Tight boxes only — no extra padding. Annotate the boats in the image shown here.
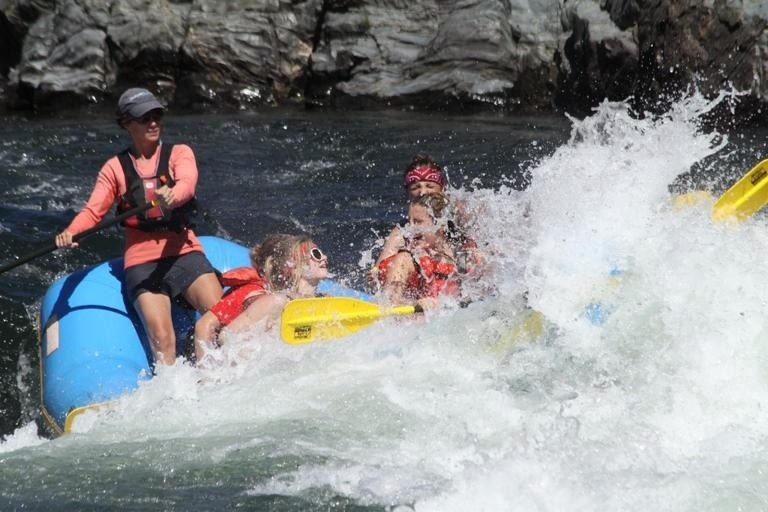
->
[36,236,379,444]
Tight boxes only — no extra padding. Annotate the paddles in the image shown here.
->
[710,156,768,230]
[280,297,483,346]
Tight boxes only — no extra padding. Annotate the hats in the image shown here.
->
[117,87,165,125]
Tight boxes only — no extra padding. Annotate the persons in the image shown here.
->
[55,87,224,366]
[372,153,486,315]
[193,234,334,361]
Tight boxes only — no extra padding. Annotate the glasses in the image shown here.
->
[309,248,324,262]
[129,109,164,125]
